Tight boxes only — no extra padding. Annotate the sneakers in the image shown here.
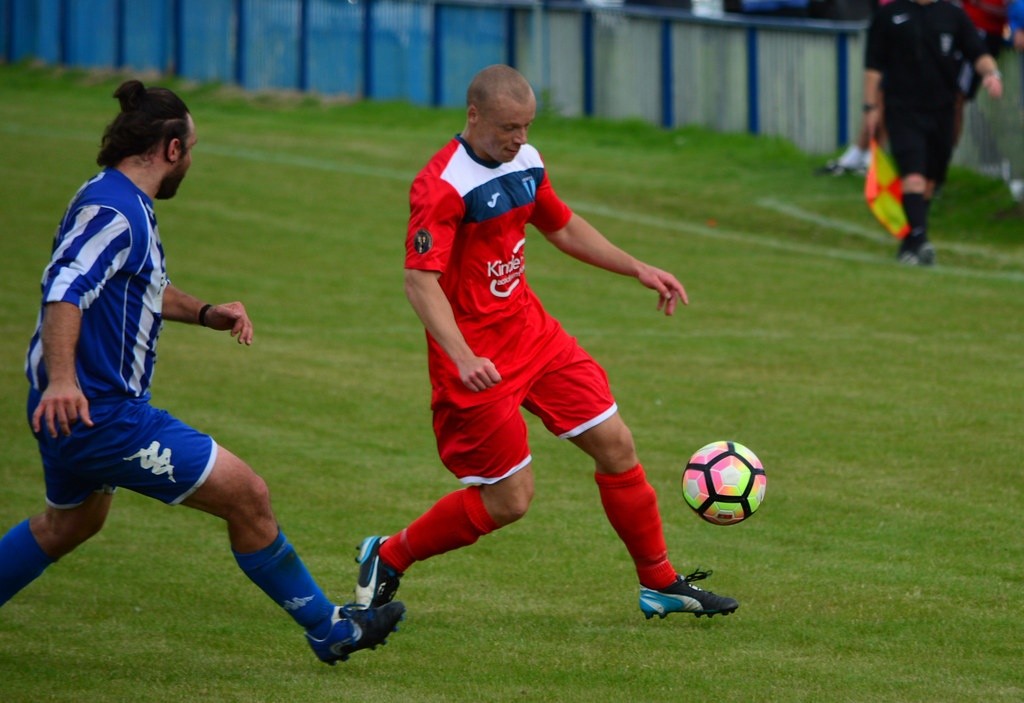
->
[354,536,403,610]
[637,574,740,619]
[304,600,408,667]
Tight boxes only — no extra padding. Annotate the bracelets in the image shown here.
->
[863,105,873,111]
[199,305,212,326]
[993,70,1002,79]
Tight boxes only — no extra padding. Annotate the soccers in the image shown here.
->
[681,438,768,526]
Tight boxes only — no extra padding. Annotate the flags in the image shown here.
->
[865,139,911,239]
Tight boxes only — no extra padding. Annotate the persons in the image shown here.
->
[810,0,1024,221]
[0,80,409,666]
[352,64,738,619]
[864,0,1002,265]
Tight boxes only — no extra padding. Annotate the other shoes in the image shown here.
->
[812,156,858,178]
[896,241,935,268]
